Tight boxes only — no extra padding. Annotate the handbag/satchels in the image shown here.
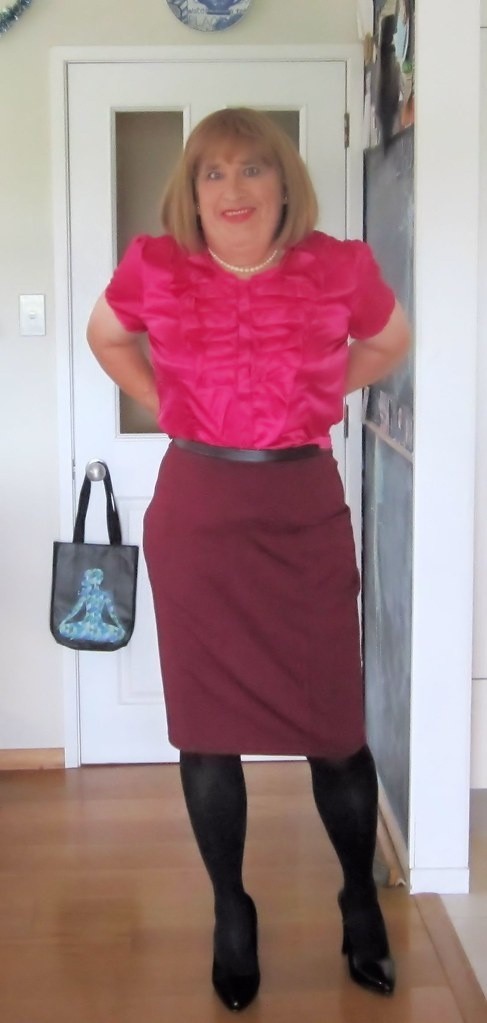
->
[51,463,139,651]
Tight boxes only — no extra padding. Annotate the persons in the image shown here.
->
[86,106,410,1012]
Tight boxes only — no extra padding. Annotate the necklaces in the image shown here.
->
[207,245,278,273]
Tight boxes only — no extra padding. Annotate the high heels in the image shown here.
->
[337,887,394,994]
[212,893,260,1013]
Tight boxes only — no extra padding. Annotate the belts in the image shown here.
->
[172,438,332,462]
